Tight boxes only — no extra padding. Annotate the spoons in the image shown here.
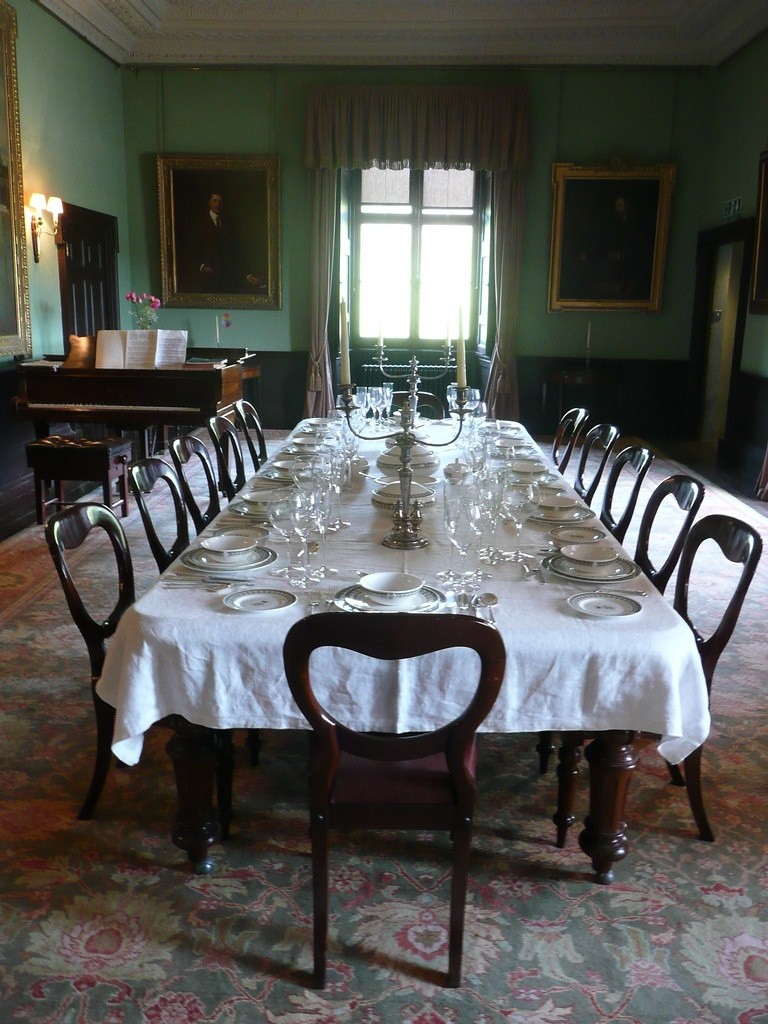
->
[481,592,499,624]
[456,590,469,615]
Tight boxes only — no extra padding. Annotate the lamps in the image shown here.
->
[30,193,64,263]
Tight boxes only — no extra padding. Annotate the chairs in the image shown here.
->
[283,612,507,991]
[44,501,261,840]
[533,407,762,849]
[375,391,445,420]
[127,400,268,575]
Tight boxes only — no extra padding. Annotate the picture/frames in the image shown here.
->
[748,150,768,316]
[155,151,282,310]
[0,0,33,362]
[547,161,675,314]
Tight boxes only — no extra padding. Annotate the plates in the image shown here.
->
[281,446,318,456]
[494,421,568,495]
[334,584,447,612]
[567,591,645,619]
[222,587,299,614]
[178,546,280,578]
[226,501,270,522]
[261,468,309,482]
[241,488,294,512]
[529,506,597,528]
[299,426,320,435]
[541,554,642,584]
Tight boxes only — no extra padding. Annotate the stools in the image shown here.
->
[26,434,135,525]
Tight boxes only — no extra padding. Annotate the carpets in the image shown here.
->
[1,432,768,1024]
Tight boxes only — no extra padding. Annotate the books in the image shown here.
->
[183,357,228,368]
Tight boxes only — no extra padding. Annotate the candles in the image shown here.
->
[586,320,591,348]
[341,296,351,385]
[444,332,451,346]
[378,331,383,346]
[216,315,219,343]
[456,303,466,388]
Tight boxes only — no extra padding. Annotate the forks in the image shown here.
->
[326,584,339,612]
[471,594,484,620]
[573,583,647,596]
[309,583,322,615]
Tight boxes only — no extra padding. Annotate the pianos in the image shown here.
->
[14,346,256,499]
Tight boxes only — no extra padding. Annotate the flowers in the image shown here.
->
[125,291,161,330]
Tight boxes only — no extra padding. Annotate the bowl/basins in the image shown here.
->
[560,544,622,574]
[374,474,441,486]
[510,462,548,482]
[527,494,579,519]
[358,571,425,606]
[548,524,607,550]
[200,535,258,563]
[307,417,328,431]
[214,525,271,548]
[292,438,324,452]
[272,459,310,477]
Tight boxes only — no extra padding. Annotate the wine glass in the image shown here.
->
[267,488,307,578]
[434,382,542,593]
[288,382,393,589]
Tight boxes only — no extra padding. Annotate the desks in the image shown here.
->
[242,364,262,425]
[96,418,711,885]
[542,368,628,438]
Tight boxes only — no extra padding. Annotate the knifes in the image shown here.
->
[164,573,254,582]
